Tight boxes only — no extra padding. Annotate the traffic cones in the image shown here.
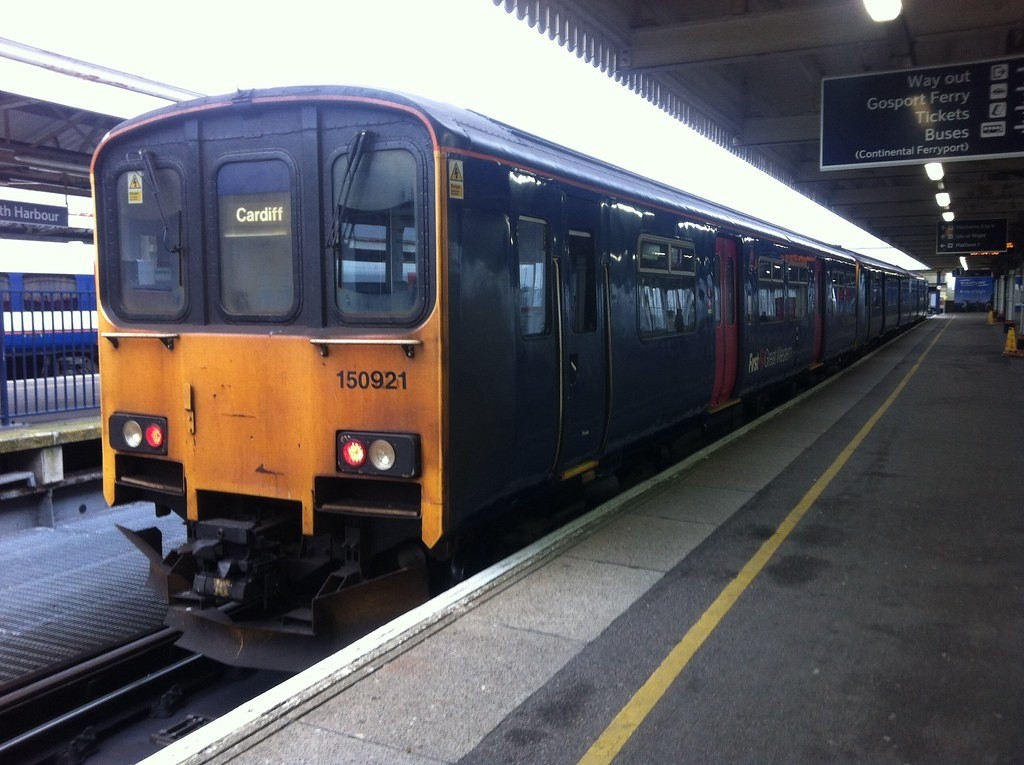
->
[1002,327,1018,357]
[987,310,994,325]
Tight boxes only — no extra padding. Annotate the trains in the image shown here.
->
[90,82,929,676]
